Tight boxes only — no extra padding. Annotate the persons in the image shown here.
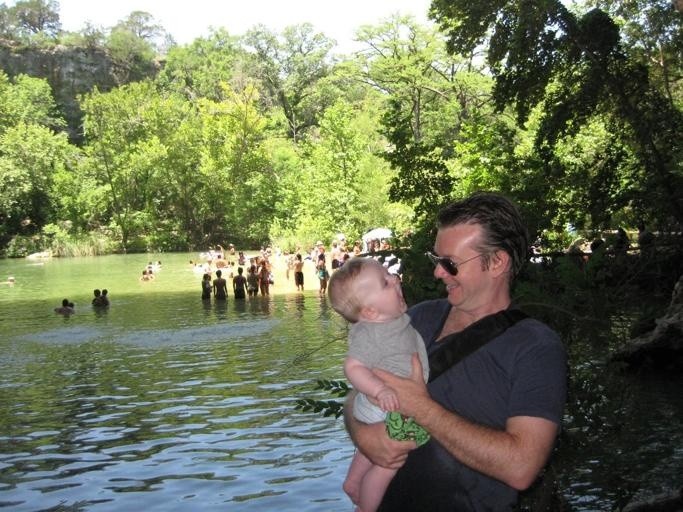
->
[341,191,569,512]
[561,224,666,277]
[325,255,429,511]
[54,298,75,315]
[139,231,410,301]
[92,289,108,307]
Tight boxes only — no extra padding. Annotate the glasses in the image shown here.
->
[426,252,485,276]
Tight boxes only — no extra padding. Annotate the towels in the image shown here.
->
[385,413,431,449]
